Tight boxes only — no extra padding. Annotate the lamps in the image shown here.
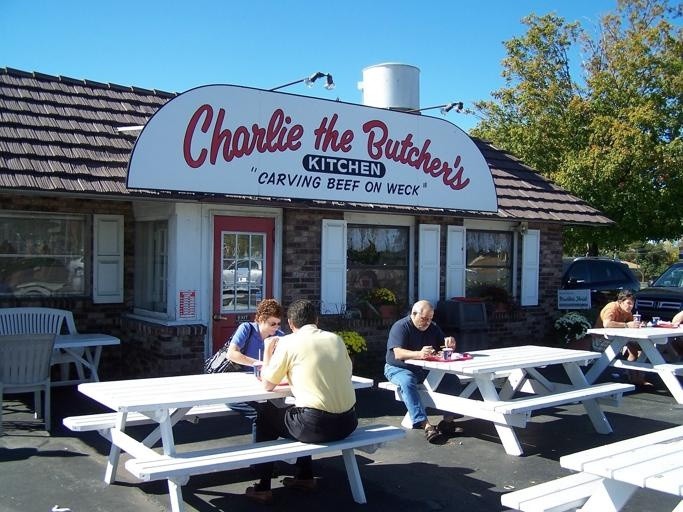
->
[404,100,466,116]
[268,68,337,94]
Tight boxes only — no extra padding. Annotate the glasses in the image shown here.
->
[269,322,282,326]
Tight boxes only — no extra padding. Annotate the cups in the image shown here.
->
[632,314,641,322]
[653,317,659,324]
[252,361,261,377]
[442,347,452,359]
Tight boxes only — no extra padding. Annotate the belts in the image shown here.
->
[304,406,356,419]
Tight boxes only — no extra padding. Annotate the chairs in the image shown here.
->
[0,330,59,434]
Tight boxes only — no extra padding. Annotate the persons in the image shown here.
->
[223,299,298,477]
[245,298,358,501]
[672,311,683,358]
[592,291,646,385]
[384,300,464,444]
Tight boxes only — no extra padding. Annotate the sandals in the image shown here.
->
[424,426,439,442]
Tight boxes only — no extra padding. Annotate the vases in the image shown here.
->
[347,349,358,370]
[367,303,399,321]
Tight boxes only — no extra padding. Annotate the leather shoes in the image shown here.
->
[245,487,272,504]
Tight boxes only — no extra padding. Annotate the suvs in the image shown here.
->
[561,257,640,312]
[629,262,682,354]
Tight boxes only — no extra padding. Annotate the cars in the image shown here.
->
[221,257,263,293]
[346,264,403,293]
[0,241,84,297]
[467,250,512,294]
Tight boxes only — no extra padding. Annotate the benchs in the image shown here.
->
[62,394,297,486]
[123,421,407,512]
[378,366,569,430]
[494,471,612,509]
[488,382,646,457]
[0,306,99,387]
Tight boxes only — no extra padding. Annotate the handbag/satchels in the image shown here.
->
[203,337,241,373]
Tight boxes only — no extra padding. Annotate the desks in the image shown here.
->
[554,420,682,508]
[398,343,616,457]
[76,366,374,512]
[49,333,120,395]
[571,326,681,406]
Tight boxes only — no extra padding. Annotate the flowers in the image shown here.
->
[331,330,367,353]
[368,286,400,305]
[553,311,592,343]
